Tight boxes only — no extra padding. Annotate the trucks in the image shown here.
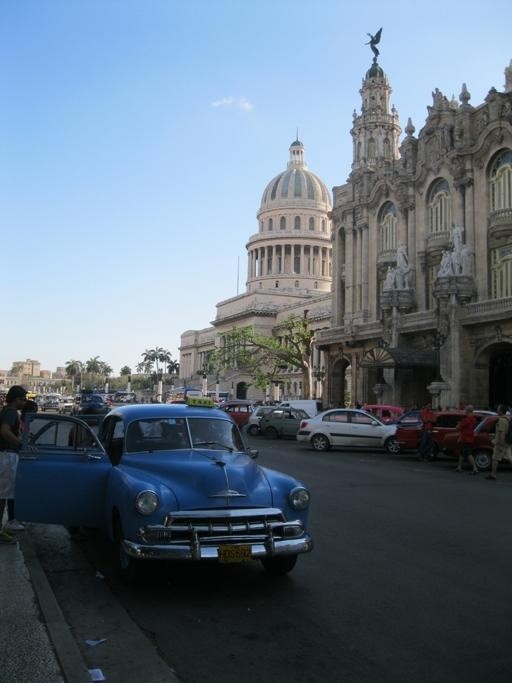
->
[259,398,323,439]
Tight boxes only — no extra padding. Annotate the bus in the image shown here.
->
[186,390,229,401]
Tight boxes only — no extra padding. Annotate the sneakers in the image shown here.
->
[1,519,23,542]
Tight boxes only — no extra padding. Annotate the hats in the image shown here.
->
[6,385,27,402]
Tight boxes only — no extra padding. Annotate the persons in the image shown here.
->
[384,223,475,290]
[364,26,384,58]
[404,116,416,137]
[254,398,512,482]
[432,86,443,110]
[17,401,38,527]
[1,384,27,544]
[459,83,471,106]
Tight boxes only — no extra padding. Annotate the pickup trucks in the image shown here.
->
[393,411,497,460]
[325,404,422,426]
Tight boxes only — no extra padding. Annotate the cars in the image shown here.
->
[297,408,402,455]
[167,400,186,404]
[216,399,255,428]
[14,396,314,577]
[0,387,136,413]
[244,405,276,435]
[259,407,311,439]
[442,413,512,471]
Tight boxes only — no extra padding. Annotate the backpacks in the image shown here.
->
[502,416,512,443]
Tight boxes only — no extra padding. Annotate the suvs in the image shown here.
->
[70,392,112,426]
